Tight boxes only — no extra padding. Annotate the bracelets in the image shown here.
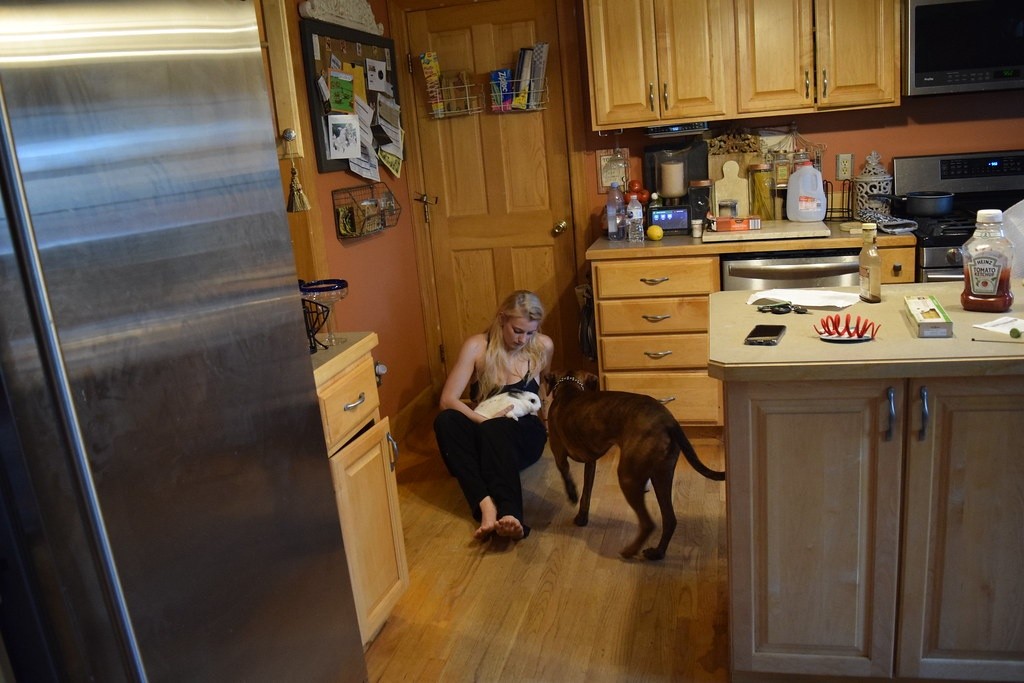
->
[543,419,548,421]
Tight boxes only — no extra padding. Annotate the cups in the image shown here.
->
[654,146,690,199]
[691,219,703,238]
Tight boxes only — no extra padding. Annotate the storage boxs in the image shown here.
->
[903,295,953,337]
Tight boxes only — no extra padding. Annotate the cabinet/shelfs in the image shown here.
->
[709,286,1024,682]
[733,1,894,114]
[588,0,724,124]
[594,253,719,430]
[315,351,408,642]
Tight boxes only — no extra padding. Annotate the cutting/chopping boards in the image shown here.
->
[701,219,831,242]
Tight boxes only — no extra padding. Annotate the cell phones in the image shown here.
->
[744,324,787,346]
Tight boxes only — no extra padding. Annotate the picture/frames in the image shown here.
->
[595,147,631,194]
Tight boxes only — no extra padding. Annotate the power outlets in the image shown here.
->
[837,153,854,181]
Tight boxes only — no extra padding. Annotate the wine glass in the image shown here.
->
[298,278,349,346]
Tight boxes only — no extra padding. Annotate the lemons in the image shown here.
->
[646,225,664,241]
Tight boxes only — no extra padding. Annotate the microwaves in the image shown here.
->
[901,0,1024,99]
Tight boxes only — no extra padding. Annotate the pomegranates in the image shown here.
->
[624,180,649,202]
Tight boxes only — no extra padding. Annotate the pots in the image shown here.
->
[868,191,955,217]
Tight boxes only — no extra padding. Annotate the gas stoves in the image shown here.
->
[892,149,1024,268]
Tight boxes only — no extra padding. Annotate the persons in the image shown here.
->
[433,289,554,540]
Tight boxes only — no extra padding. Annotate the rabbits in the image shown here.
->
[474,388,542,422]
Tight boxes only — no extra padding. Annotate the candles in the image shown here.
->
[661,161,685,197]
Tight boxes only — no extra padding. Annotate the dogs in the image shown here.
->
[544,368,729,561]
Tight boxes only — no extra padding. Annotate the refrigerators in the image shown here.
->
[0,3,370,681]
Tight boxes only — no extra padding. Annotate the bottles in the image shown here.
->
[626,195,644,243]
[748,163,777,219]
[859,223,882,304]
[961,209,1014,313]
[772,149,810,188]
[607,182,626,241]
[786,162,827,223]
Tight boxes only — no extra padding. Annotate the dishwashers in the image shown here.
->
[720,253,859,290]
